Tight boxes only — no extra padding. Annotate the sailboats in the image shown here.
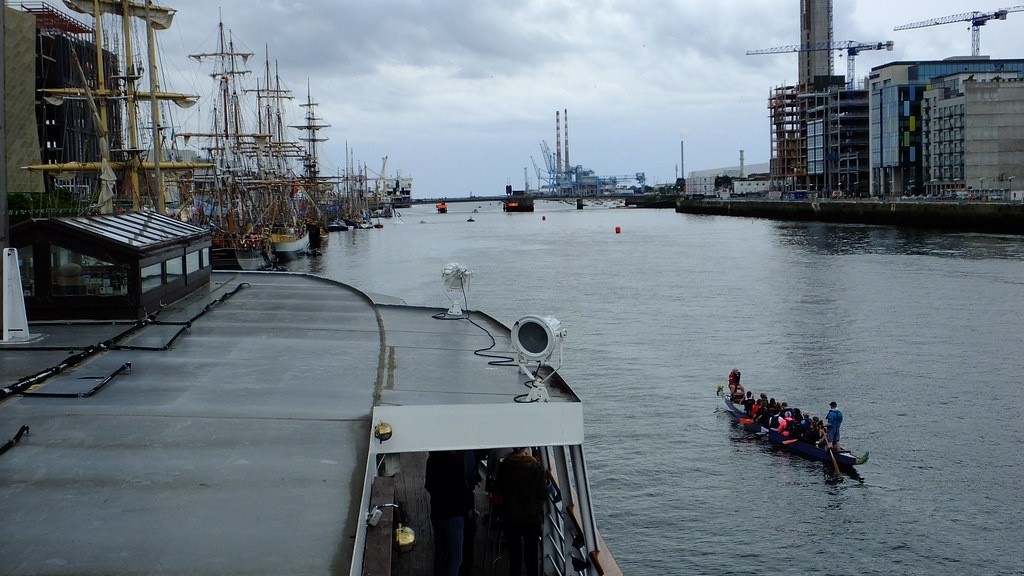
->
[19,0,415,271]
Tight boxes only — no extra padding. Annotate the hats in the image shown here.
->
[829,402,837,407]
[732,367,739,371]
[803,413,809,416]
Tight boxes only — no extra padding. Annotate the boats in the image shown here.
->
[715,385,869,473]
[498,189,536,213]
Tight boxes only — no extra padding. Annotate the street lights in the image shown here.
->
[1008,176,1017,202]
[980,177,987,198]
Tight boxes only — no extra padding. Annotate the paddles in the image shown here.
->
[781,438,799,444]
[822,428,840,473]
[739,418,757,425]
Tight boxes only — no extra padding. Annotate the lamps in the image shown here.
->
[441,263,473,315]
[511,315,568,403]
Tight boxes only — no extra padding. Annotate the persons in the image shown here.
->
[728,368,745,401]
[739,390,825,449]
[825,402,843,450]
[423,450,483,576]
[212,230,263,248]
[491,447,550,576]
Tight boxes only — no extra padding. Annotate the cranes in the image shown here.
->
[746,39,895,91]
[893,5,1024,57]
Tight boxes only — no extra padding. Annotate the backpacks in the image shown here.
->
[783,419,795,432]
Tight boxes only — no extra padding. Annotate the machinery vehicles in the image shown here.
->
[529,140,647,198]
